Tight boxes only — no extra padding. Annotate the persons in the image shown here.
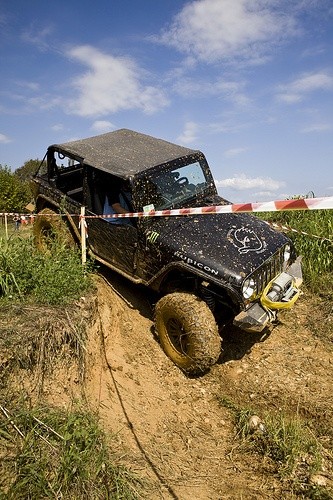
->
[102,176,134,228]
[13,215,19,231]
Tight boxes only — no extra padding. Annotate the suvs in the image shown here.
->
[29,127,305,379]
